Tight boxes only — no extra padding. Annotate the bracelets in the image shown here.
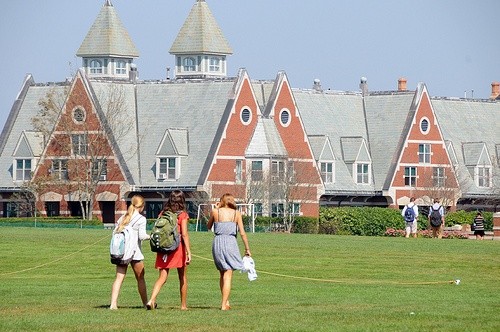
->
[245,248,249,251]
[187,251,191,255]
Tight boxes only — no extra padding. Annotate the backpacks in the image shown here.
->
[149,206,184,253]
[405,204,415,222]
[109,212,143,267]
[430,205,442,227]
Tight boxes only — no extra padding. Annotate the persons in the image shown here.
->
[145,190,192,311]
[401,197,419,238]
[108,195,158,311]
[207,193,252,311]
[427,198,444,239]
[474,210,485,240]
